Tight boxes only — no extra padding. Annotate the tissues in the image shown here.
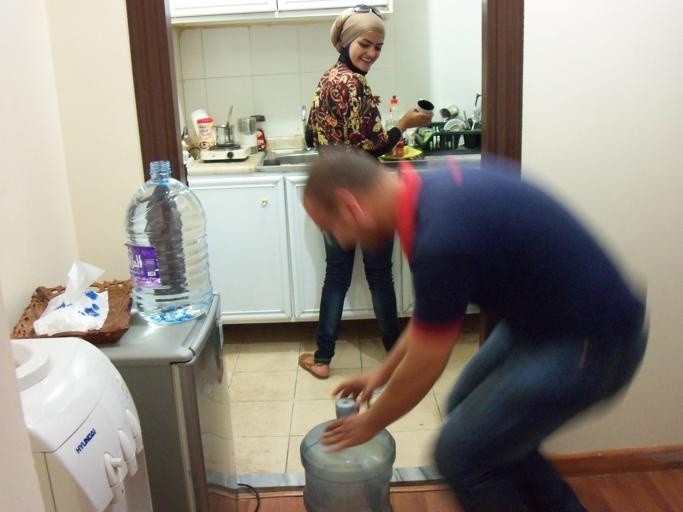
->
[31,257,109,336]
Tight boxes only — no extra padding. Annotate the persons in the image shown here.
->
[298,4,434,379]
[300,146,648,511]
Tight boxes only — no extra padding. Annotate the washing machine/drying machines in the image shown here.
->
[11,335,155,512]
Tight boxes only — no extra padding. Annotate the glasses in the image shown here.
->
[348,4,385,20]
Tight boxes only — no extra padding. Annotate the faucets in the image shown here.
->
[300,105,312,152]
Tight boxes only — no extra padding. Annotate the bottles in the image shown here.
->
[385,96,401,131]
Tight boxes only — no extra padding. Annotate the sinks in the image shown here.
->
[254,147,320,171]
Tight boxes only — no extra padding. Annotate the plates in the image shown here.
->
[380,145,422,161]
[444,119,469,132]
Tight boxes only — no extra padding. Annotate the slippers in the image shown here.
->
[298,353,329,379]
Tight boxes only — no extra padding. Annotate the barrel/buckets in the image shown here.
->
[122,158,215,324]
[298,397,397,512]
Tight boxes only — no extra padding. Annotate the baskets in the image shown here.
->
[11,278,133,346]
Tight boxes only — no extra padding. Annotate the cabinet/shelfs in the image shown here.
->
[169,0,394,29]
[285,173,481,322]
[188,175,293,325]
